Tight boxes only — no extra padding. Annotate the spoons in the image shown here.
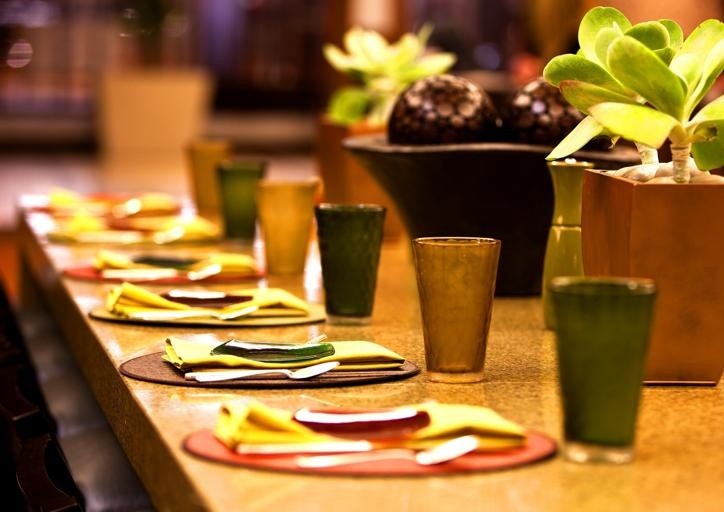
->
[125,305,260,322]
[296,433,479,468]
[195,359,339,383]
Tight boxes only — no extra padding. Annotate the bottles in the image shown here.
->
[540,160,594,331]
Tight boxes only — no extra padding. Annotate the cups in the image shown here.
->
[256,177,320,274]
[548,276,658,461]
[413,237,502,382]
[215,155,267,239]
[187,140,227,217]
[314,203,388,323]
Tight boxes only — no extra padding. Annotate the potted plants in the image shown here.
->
[316,27,453,242]
[546,6,724,387]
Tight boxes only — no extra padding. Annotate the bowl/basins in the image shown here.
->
[340,133,643,298]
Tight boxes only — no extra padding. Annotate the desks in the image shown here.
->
[1,192,724,512]
